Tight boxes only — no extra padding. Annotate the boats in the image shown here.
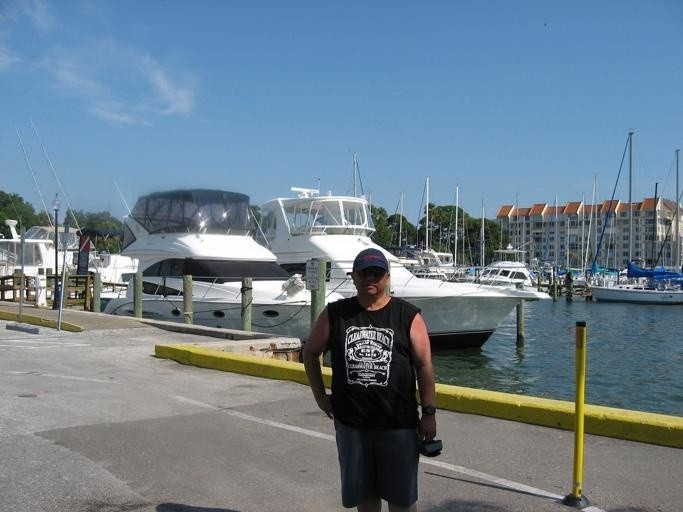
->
[0,218,137,301]
[103,191,345,345]
[258,194,538,346]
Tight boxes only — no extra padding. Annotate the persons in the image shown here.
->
[301,247,436,512]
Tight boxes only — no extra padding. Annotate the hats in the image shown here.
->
[353,249,388,272]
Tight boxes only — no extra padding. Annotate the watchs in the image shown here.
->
[421,405,436,416]
